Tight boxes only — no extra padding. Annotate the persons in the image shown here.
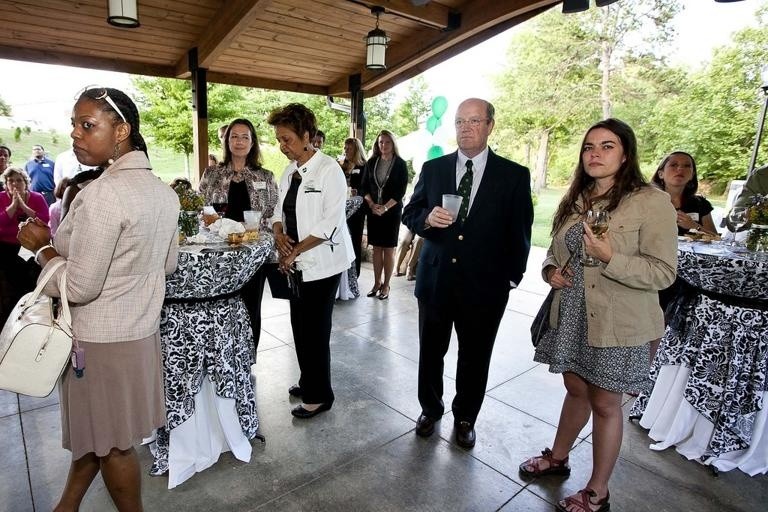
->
[0,146,12,192]
[312,130,325,151]
[59,164,103,224]
[267,101,358,418]
[17,87,182,512]
[23,145,56,208]
[197,119,278,369]
[627,151,721,397]
[208,155,218,167]
[47,176,71,243]
[518,117,678,512]
[341,137,369,281]
[393,229,425,282]
[402,99,535,450]
[728,164,768,233]
[218,125,228,145]
[360,129,409,300]
[0,166,49,330]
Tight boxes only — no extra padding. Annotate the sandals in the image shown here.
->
[519,448,571,479]
[556,487,610,512]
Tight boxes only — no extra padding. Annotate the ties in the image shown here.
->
[457,160,473,223]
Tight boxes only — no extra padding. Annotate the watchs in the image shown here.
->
[696,225,700,231]
[383,205,388,212]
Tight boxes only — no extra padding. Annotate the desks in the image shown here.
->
[627,240,767,478]
[158,222,275,445]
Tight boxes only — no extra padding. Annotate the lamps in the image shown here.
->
[361,5,392,71]
[106,0,141,28]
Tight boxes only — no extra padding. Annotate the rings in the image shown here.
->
[679,220,682,222]
[281,266,285,269]
[277,268,281,270]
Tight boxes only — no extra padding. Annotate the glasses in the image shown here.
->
[74,84,126,122]
[455,118,488,126]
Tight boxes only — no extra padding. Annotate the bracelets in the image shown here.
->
[35,245,56,265]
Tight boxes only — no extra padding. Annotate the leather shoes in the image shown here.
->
[289,385,301,396]
[416,413,442,437]
[454,418,475,448]
[291,400,334,419]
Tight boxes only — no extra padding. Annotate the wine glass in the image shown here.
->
[728,206,749,245]
[578,208,609,267]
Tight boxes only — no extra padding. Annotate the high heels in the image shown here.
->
[379,287,390,300]
[367,283,384,297]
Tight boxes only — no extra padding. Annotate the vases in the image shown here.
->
[743,223,767,253]
[181,210,200,243]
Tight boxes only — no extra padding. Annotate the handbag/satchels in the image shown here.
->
[531,288,555,347]
[0,261,85,399]
[266,270,305,299]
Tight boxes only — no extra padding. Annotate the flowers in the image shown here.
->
[173,184,208,236]
[747,194,767,251]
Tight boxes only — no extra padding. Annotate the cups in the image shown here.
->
[442,194,463,223]
[243,211,261,242]
